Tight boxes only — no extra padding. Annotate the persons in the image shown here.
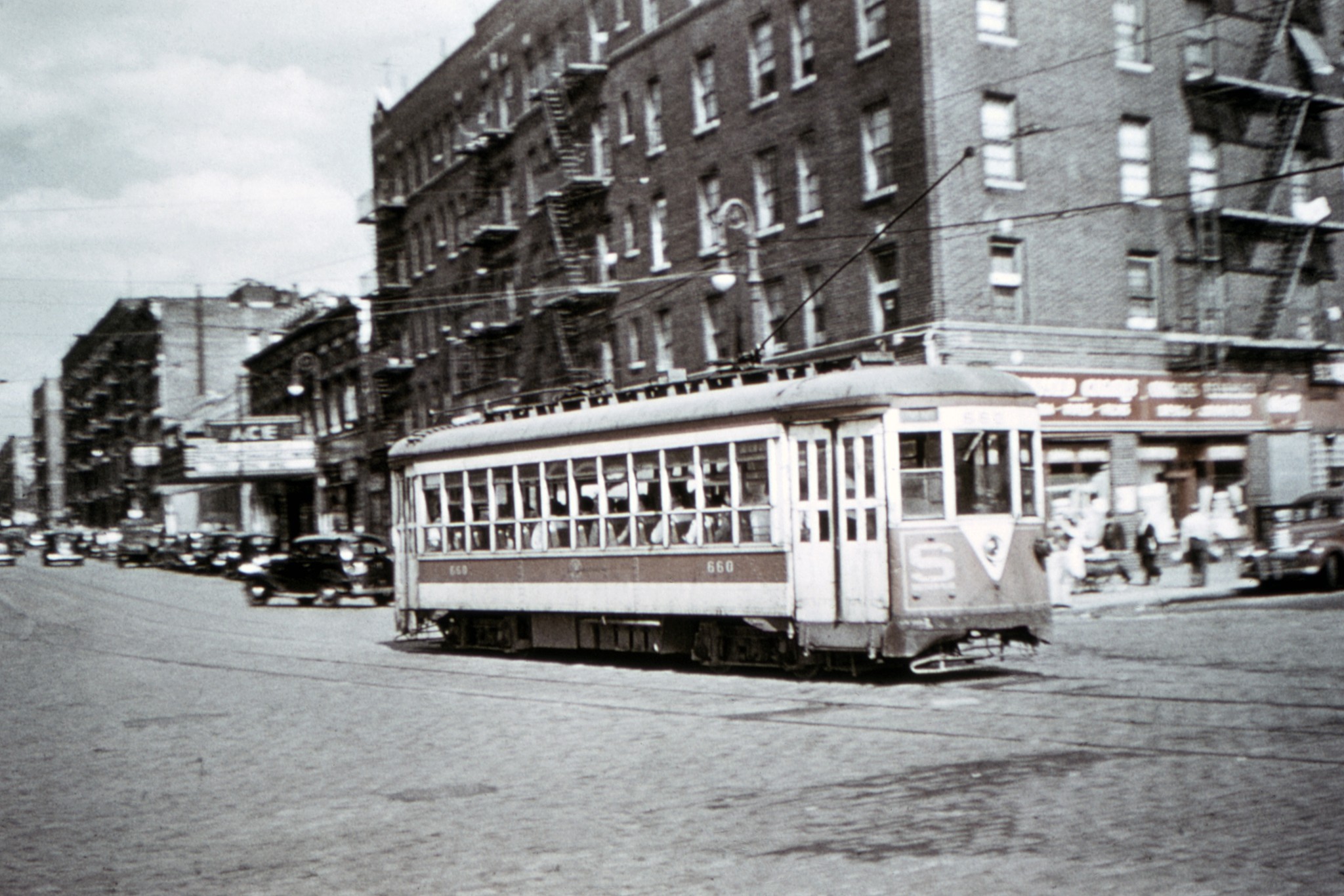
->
[1179,503,1218,587]
[1133,510,1162,582]
[649,481,693,543]
[426,494,658,552]
[682,483,716,545]
[955,471,970,514]
[1097,510,1131,585]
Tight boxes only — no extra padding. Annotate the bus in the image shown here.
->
[387,145,1056,680]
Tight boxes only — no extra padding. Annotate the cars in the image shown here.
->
[238,534,395,609]
[1239,488,1344,590]
[0,522,282,581]
